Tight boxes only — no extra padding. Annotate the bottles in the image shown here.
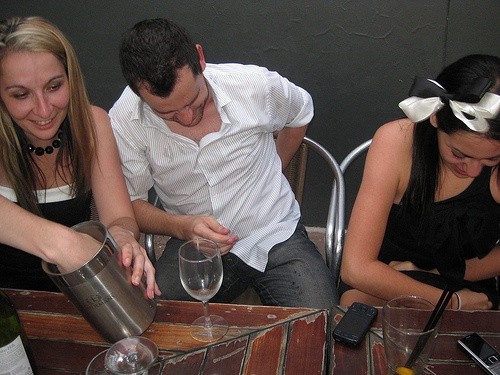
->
[0,289,39,375]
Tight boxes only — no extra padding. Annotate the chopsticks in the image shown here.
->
[403,288,455,370]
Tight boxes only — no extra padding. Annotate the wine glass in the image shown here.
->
[103,336,159,375]
[178,237,228,343]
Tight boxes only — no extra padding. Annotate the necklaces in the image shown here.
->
[22,128,67,158]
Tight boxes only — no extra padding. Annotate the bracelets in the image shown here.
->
[453,292,461,310]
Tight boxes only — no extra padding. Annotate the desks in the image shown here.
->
[326,304,500,375]
[0,288,329,375]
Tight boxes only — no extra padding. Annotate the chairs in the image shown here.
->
[324,139,373,270]
[144,137,344,304]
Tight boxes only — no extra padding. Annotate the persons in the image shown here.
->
[1,15,162,301]
[338,54,499,309]
[107,18,338,311]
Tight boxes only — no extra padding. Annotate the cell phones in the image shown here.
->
[332,302,378,349]
[456,332,500,375]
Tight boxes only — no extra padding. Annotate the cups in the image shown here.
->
[382,295,443,375]
[41,219,156,344]
